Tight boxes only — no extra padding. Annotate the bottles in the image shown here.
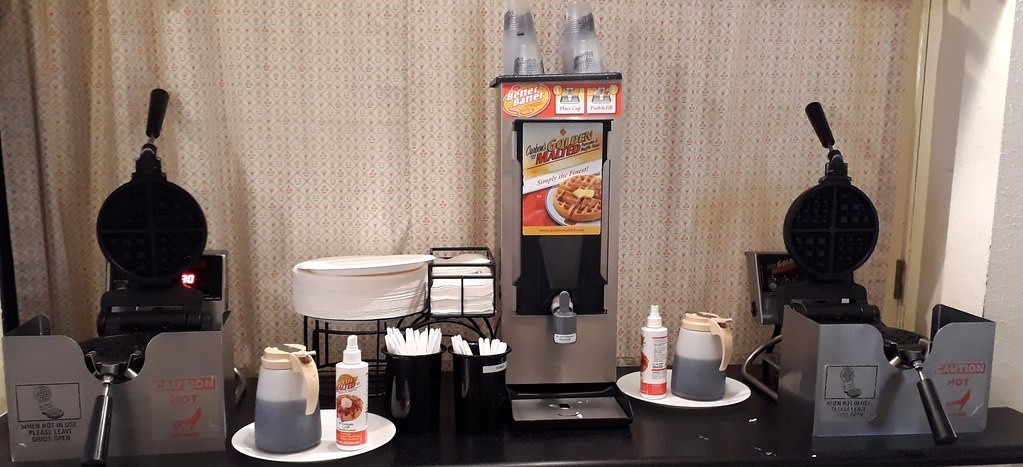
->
[335,335,369,450]
[640,305,668,399]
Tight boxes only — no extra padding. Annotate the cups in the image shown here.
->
[500,0,543,78]
[448,343,512,434]
[561,5,604,75]
[381,342,449,431]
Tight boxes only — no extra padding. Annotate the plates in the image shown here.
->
[291,254,434,321]
[232,410,396,462]
[546,185,601,226]
[616,370,751,408]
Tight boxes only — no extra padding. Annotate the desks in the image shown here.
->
[0,364,1023,467]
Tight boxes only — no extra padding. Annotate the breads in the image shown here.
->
[554,174,601,222]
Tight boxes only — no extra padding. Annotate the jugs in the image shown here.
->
[255,344,322,454]
[670,313,732,400]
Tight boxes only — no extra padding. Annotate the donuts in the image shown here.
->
[640,351,649,372]
[335,393,362,421]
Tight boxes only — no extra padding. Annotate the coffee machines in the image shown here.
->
[489,72,630,425]
